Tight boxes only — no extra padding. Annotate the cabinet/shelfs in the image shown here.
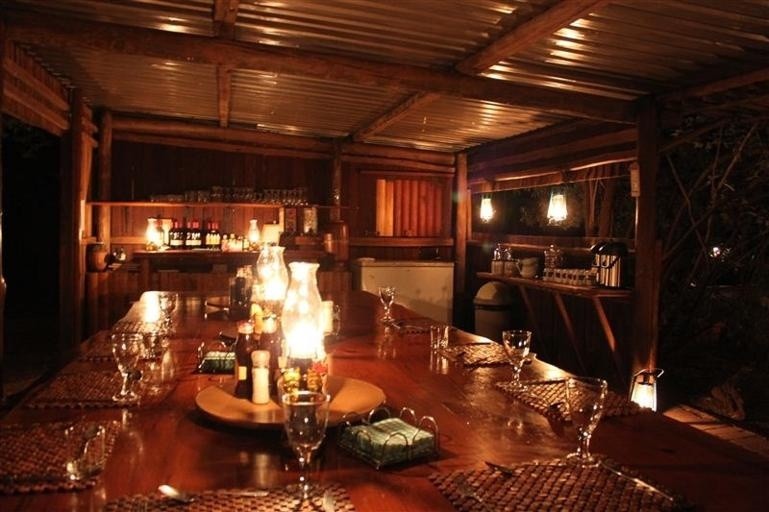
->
[477,270,635,394]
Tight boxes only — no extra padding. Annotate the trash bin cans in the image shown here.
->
[473,281,515,346]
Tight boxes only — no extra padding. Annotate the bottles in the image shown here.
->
[221,232,249,253]
[491,242,593,287]
[249,217,260,254]
[168,220,221,249]
[232,313,281,407]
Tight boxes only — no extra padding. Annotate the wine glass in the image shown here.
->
[377,286,397,323]
[501,393,527,453]
[563,374,609,470]
[150,185,310,204]
[499,327,532,393]
[110,283,177,403]
[283,392,334,499]
[378,324,397,361]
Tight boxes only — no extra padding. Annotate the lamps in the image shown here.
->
[545,179,569,229]
[477,186,496,222]
[280,260,344,458]
[246,243,290,364]
[629,363,667,414]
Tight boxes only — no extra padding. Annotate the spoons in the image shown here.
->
[159,483,268,502]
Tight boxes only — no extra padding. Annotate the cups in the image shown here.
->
[64,423,107,479]
[429,323,450,349]
[331,306,341,334]
[430,351,450,375]
[65,478,109,511]
